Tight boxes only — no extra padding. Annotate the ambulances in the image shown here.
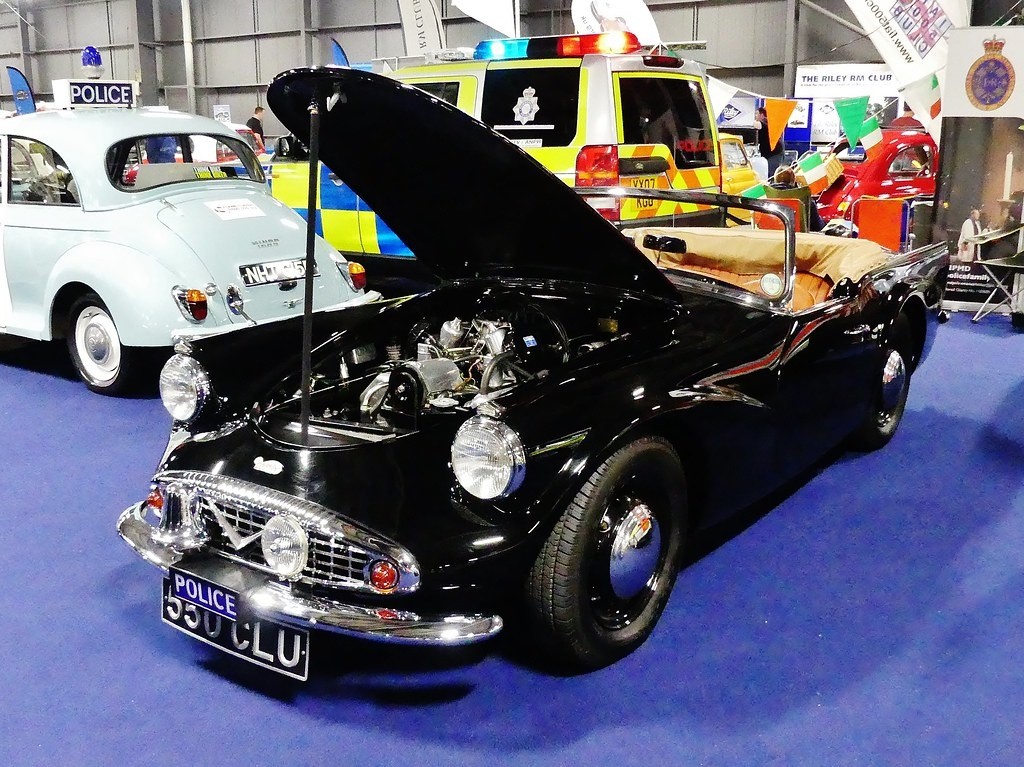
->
[199,32,723,265]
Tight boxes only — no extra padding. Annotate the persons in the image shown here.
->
[754,107,785,181]
[770,166,825,232]
[891,101,925,173]
[983,220,992,233]
[958,210,980,262]
[246,107,265,152]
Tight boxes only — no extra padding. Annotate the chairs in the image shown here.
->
[750,183,812,234]
[964,220,1024,326]
[849,195,915,256]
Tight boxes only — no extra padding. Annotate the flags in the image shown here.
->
[931,73,941,120]
[859,116,884,161]
[742,184,768,199]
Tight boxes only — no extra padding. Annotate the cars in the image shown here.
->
[812,130,941,230]
[716,133,761,226]
[139,122,265,163]
[117,64,950,686]
[0,46,384,397]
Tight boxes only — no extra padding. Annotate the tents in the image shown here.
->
[799,152,828,194]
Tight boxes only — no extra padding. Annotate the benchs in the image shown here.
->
[621,226,889,311]
